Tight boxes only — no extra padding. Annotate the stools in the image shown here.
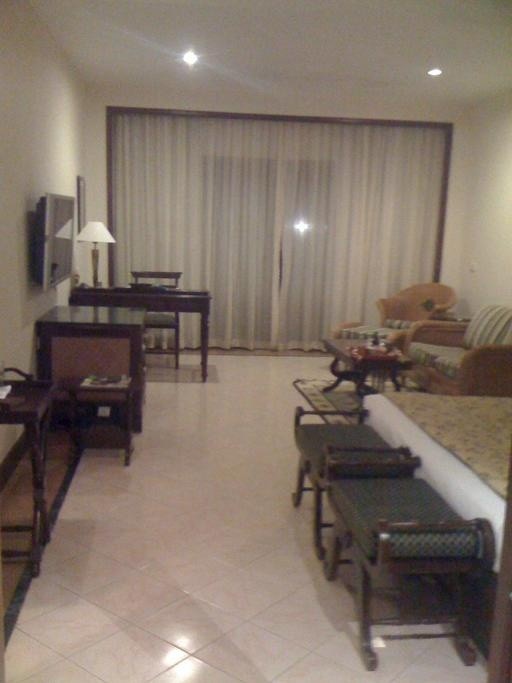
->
[334,321,408,350]
[78,377,140,467]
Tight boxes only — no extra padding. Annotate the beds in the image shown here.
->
[366,392,510,572]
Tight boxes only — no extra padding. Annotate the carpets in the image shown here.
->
[293,380,416,424]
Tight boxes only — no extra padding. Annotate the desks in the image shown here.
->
[37,305,144,434]
[72,285,213,382]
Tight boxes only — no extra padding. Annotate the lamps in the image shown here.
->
[76,221,117,289]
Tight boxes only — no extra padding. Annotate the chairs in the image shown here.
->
[129,271,183,369]
[376,280,512,392]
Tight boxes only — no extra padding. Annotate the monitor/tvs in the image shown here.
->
[33,192,77,293]
